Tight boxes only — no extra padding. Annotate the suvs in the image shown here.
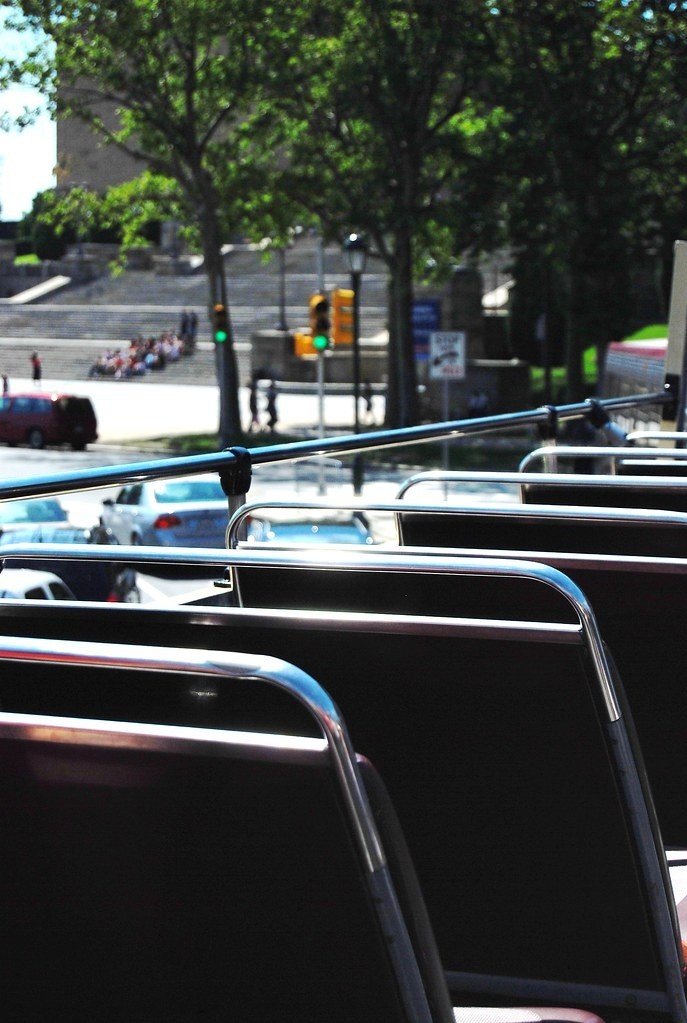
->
[0,497,141,600]
[249,511,376,546]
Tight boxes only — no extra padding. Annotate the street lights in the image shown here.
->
[341,232,369,433]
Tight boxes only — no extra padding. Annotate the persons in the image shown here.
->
[361,379,376,424]
[89,308,197,379]
[247,380,278,432]
[32,351,42,380]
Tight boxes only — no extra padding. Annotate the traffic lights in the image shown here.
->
[310,295,331,353]
[331,288,355,346]
[211,303,230,345]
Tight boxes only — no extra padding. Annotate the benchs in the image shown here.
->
[0,432,686,1023]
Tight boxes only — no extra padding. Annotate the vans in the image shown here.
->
[0,393,98,449]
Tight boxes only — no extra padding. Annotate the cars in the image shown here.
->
[99,476,252,571]
